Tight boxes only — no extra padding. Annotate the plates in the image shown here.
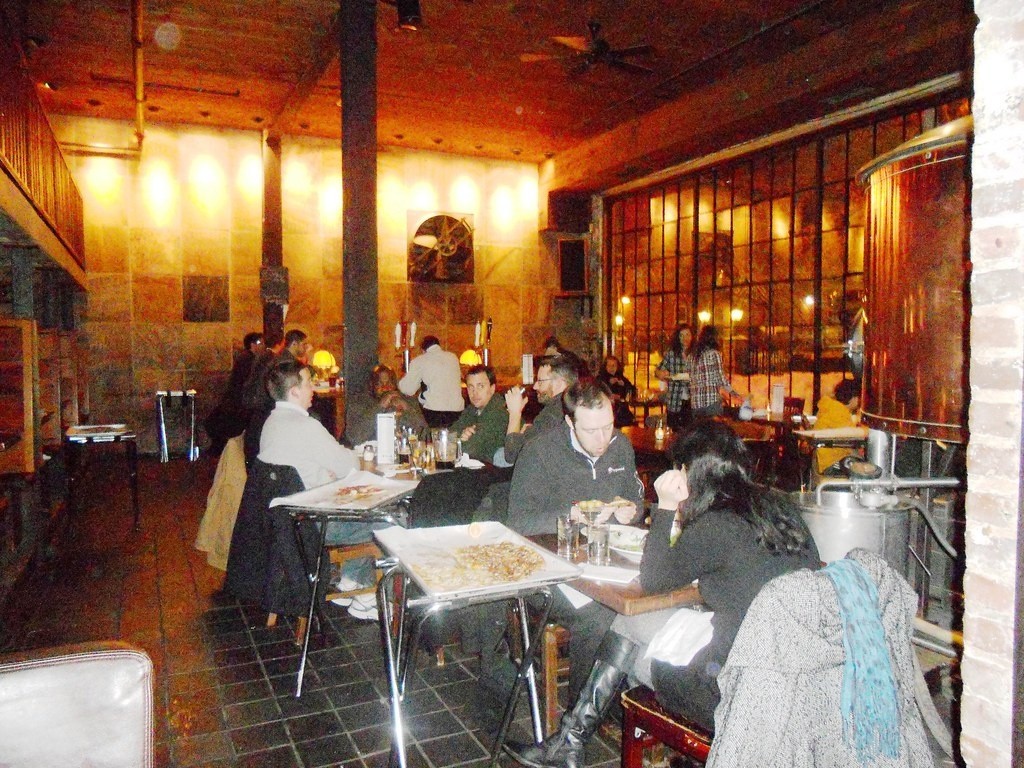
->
[581,524,641,541]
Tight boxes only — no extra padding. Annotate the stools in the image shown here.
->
[68,431,141,529]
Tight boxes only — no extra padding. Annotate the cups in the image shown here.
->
[432,434,457,469]
[394,441,399,461]
[313,378,318,386]
[588,523,609,564]
[329,378,336,390]
[503,386,531,412]
[409,451,428,476]
[579,507,601,523]
[558,515,579,557]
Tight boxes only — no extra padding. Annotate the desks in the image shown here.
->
[271,381,813,768]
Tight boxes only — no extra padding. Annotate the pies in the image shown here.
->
[578,495,631,512]
[404,542,545,592]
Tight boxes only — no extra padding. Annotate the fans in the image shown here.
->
[521,22,653,78]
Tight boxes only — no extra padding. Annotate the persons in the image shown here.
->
[505,423,821,768]
[507,381,644,725]
[228,325,859,621]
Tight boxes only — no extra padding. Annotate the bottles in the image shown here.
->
[340,372,344,380]
[395,422,432,463]
[341,382,344,391]
[670,521,682,547]
[364,446,374,461]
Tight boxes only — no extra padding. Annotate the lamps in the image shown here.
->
[460,349,482,365]
[314,351,335,370]
[395,0,420,31]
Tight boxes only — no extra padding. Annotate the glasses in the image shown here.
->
[536,376,553,385]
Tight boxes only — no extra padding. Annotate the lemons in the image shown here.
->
[468,522,483,537]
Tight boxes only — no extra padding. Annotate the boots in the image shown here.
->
[499,630,639,768]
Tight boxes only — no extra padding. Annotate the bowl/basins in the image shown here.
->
[610,530,650,562]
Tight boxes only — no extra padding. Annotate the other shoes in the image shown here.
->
[327,583,356,606]
[558,706,571,727]
[347,595,394,621]
[609,690,626,725]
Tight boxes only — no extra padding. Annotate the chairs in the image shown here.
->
[0,646,155,768]
[216,395,936,768]
